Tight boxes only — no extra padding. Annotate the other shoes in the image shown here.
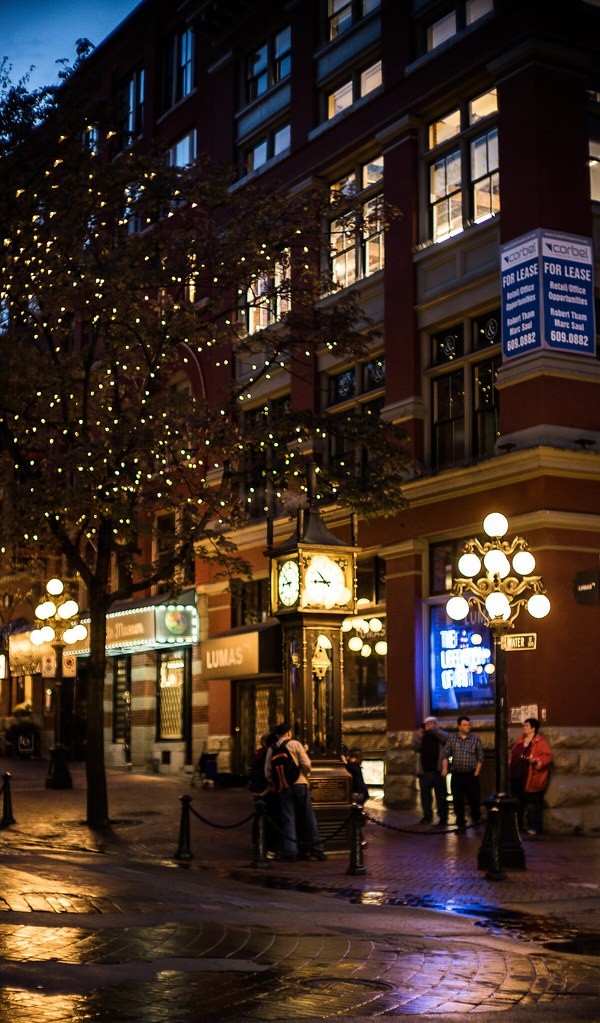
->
[421,817,432,824]
[310,850,328,860]
[438,821,447,826]
[266,850,284,860]
[527,832,535,839]
[459,825,466,830]
[283,852,298,862]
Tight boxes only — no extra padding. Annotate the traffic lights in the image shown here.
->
[500,633,537,652]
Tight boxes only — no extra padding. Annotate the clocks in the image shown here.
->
[278,561,299,606]
[305,559,345,605]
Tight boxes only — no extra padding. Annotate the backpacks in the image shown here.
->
[247,752,267,796]
[270,740,301,794]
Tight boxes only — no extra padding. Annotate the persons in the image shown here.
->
[509,718,551,835]
[442,717,484,832]
[413,717,449,828]
[341,745,368,848]
[249,723,327,861]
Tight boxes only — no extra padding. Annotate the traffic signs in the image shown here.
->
[447,514,552,871]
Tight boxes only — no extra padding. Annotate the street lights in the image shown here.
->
[30,578,88,790]
[340,598,388,722]
